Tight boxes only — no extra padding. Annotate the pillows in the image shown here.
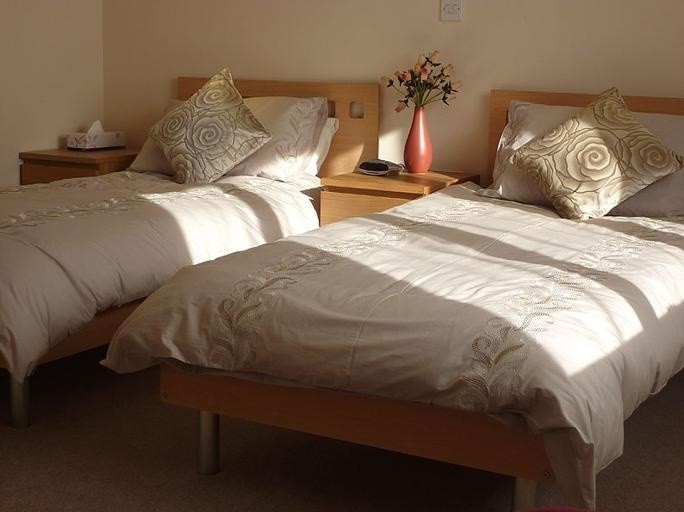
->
[515,86,684,220]
[150,66,272,184]
[305,117,338,176]
[475,100,684,221]
[126,97,328,181]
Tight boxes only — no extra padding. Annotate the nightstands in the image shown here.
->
[320,169,481,227]
[19,147,140,186]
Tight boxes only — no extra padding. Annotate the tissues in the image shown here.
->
[66,120,126,149]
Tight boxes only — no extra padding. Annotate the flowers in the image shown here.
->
[380,50,462,115]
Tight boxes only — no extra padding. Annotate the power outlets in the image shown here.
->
[438,0,464,23]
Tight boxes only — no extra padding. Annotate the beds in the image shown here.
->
[102,88,684,512]
[0,77,380,428]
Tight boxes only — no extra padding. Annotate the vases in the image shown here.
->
[403,101,434,174]
